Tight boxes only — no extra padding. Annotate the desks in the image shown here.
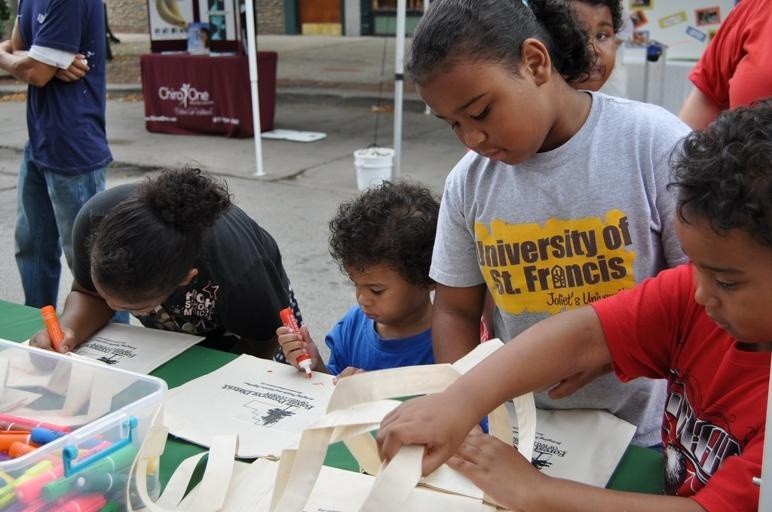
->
[140,51,278,139]
[0,299,664,512]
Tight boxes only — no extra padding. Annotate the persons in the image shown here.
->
[373,94,772,512]
[27,162,302,376]
[276,175,490,441]
[678,1,772,135]
[0,0,129,325]
[405,0,695,450]
[551,0,625,92]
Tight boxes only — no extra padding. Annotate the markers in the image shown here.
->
[1,414,154,512]
[40,304,70,354]
[279,304,313,379]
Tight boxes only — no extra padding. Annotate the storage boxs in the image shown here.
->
[0,338,168,512]
